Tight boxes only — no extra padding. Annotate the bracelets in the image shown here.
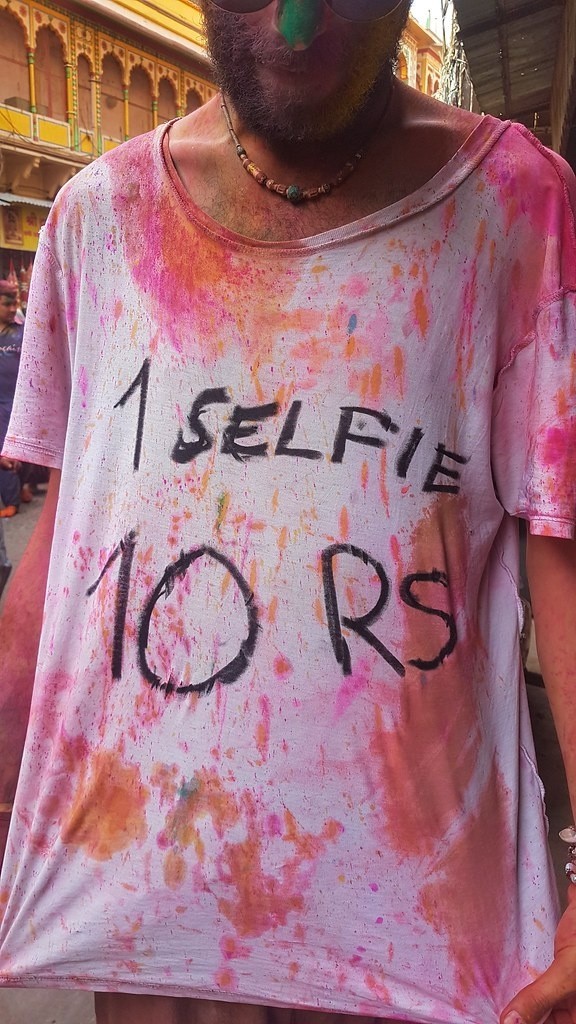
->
[559,825,576,884]
[0,803,13,813]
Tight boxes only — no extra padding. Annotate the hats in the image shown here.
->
[0,279,17,297]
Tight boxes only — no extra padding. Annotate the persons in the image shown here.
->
[0,0,576,1024]
[0,279,52,518]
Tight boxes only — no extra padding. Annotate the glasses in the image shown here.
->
[211,0,401,24]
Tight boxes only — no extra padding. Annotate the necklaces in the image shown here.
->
[215,88,391,203]
